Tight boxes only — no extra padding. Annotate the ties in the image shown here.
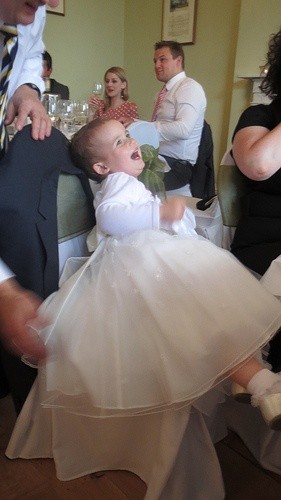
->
[0,23,18,152]
[152,86,168,120]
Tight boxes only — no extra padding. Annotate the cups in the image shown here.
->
[42,94,60,122]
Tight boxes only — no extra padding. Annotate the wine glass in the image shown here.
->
[59,101,76,131]
[75,104,88,127]
[92,83,102,97]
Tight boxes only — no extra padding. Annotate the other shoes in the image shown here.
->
[230,381,252,405]
[259,393,281,429]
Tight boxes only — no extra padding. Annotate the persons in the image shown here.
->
[5,118,281,500]
[41,50,69,100]
[132,40,207,191]
[89,66,138,127]
[0,0,60,417]
[227,26,281,373]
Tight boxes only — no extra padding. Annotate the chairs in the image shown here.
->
[161,120,215,202]
[217,148,239,250]
[0,123,98,299]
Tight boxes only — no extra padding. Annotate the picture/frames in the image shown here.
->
[46,0,66,16]
[161,0,197,45]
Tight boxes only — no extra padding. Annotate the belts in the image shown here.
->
[176,159,192,168]
